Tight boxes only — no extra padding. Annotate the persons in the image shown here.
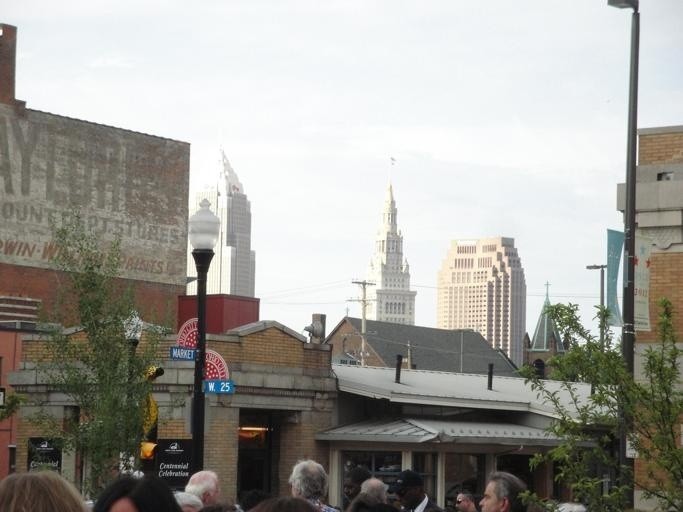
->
[171,460,342,512]
[90,476,183,512]
[454,469,585,512]
[1,471,90,512]
[342,468,444,512]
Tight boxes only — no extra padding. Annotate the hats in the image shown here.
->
[388,469,424,494]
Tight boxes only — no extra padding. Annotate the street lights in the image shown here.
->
[182,199,224,481]
[585,264,606,378]
[117,309,145,475]
[599,0,641,511]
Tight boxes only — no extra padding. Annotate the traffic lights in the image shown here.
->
[136,360,163,464]
